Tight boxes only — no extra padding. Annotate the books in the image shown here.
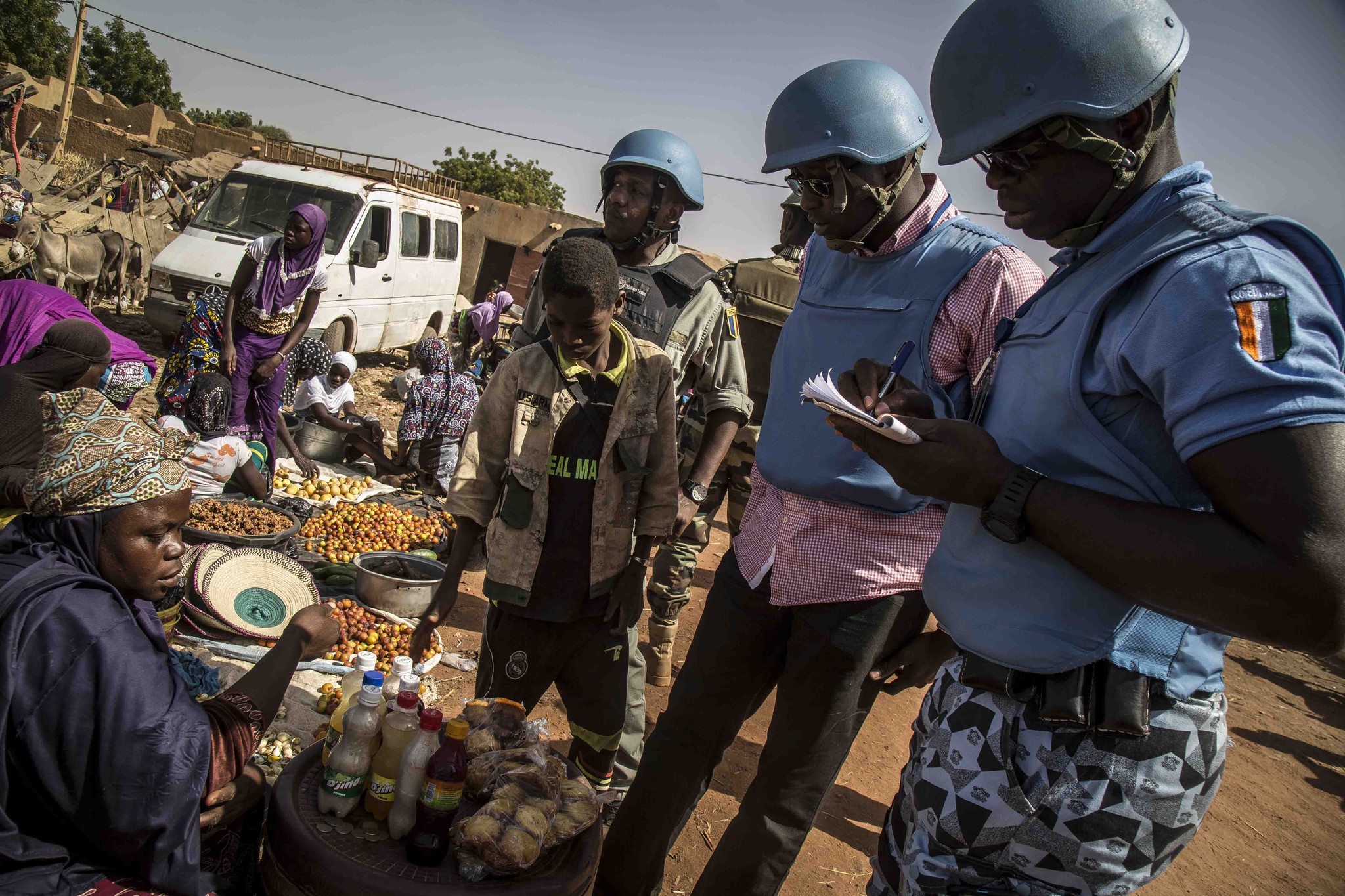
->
[799,367,923,445]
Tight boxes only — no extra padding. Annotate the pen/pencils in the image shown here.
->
[867,340,917,417]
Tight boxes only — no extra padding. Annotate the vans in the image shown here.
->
[145,136,463,372]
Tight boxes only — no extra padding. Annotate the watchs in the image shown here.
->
[629,554,650,568]
[682,478,710,504]
[979,463,1048,544]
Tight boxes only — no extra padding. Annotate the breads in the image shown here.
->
[457,697,597,880]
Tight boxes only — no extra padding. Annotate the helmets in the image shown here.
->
[780,192,801,210]
[760,59,932,174]
[929,1,1190,166]
[600,129,705,212]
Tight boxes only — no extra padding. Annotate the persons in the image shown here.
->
[143,170,207,213]
[824,0,1345,896]
[290,349,408,478]
[634,173,816,689]
[506,126,756,792]
[106,168,131,213]
[407,236,679,794]
[589,57,1048,896]
[155,373,270,504]
[154,293,334,421]
[0,278,158,411]
[1,385,342,896]
[393,332,480,498]
[445,291,515,376]
[219,203,330,481]
[0,317,114,531]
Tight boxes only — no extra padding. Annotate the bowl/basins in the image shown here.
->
[295,416,349,464]
[353,550,449,619]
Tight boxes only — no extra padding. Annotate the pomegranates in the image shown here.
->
[272,468,373,502]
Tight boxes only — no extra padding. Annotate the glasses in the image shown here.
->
[784,161,862,199]
[972,137,1050,174]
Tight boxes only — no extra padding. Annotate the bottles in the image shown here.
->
[319,648,473,868]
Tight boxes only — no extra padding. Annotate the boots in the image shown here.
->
[636,617,679,687]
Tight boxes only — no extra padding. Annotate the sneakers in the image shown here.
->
[600,799,623,842]
